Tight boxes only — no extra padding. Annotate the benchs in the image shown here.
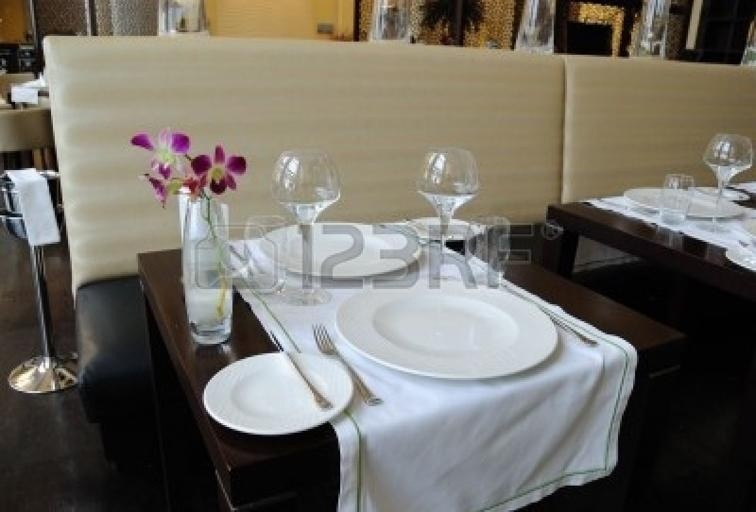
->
[41,31,756,472]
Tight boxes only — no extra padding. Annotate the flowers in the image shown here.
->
[129,123,249,325]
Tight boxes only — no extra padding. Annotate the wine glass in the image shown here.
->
[703,133,753,233]
[418,146,478,278]
[269,149,342,306]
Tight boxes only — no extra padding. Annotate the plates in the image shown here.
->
[624,185,752,219]
[725,246,756,271]
[200,220,558,437]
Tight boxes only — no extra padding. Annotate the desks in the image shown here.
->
[137,183,756,510]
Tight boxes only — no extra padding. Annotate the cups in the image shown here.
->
[181,201,232,345]
[464,215,511,289]
[657,174,694,227]
[244,215,286,295]
